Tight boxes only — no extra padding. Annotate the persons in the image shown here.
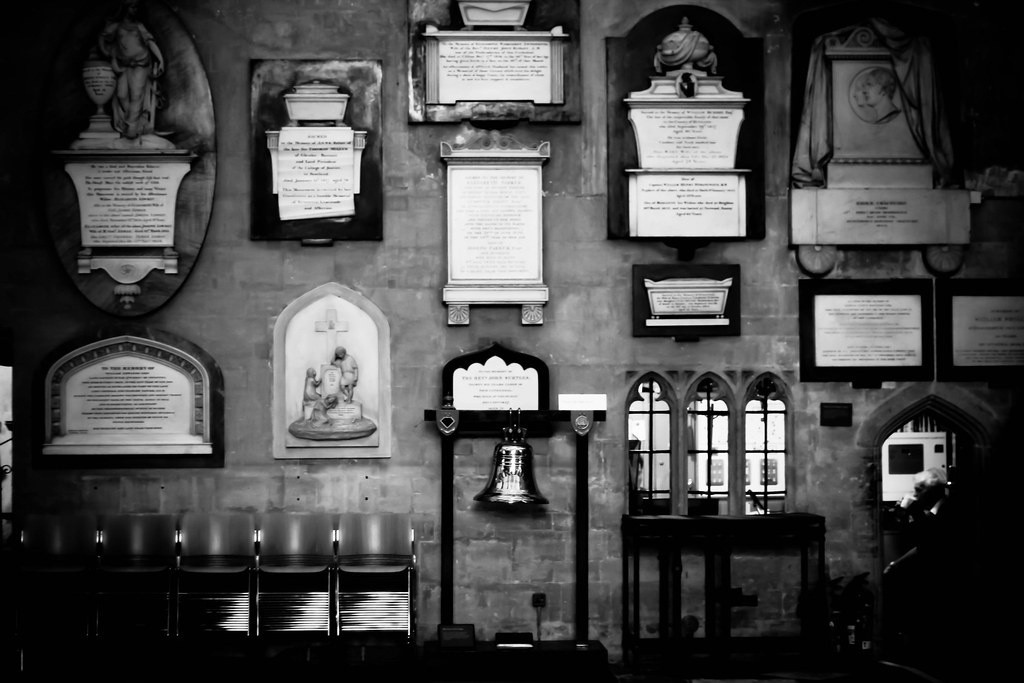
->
[98,20,162,139]
[853,67,900,123]
[883,467,977,576]
[304,346,358,426]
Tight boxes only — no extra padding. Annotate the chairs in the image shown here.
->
[6,508,417,640]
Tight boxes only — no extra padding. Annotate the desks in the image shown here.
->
[622,513,828,657]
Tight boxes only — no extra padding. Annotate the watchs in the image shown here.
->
[889,561,896,567]
